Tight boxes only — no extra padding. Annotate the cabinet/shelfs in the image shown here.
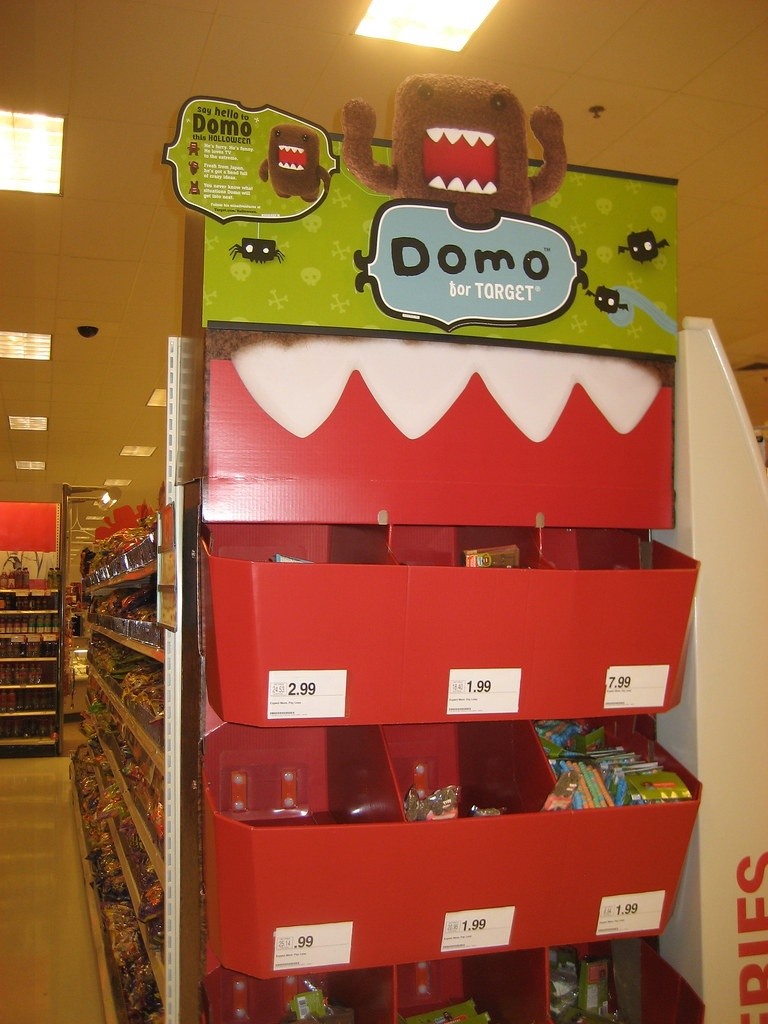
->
[63,505,167,1024]
[0,501,61,758]
[167,311,768,1023]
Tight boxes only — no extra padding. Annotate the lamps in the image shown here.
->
[99,487,122,512]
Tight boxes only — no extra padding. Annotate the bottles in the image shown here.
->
[0,663,46,685]
[48,567,59,589]
[7,567,29,589]
[0,614,58,633]
[0,572,7,589]
[0,689,56,712]
